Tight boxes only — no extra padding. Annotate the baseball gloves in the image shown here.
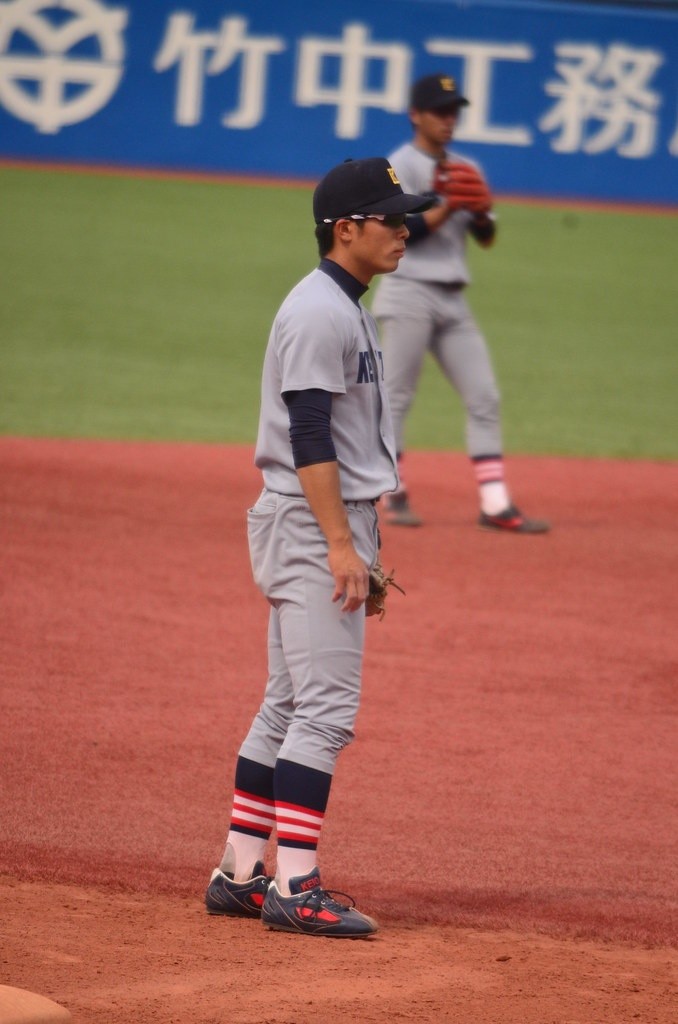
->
[365,562,388,618]
[432,160,492,213]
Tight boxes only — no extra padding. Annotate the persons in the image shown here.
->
[371,72,552,537]
[207,155,442,939]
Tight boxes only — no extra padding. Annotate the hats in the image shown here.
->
[313,157,434,224]
[412,75,469,109]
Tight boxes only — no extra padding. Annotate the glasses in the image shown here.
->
[432,108,460,119]
[324,214,407,229]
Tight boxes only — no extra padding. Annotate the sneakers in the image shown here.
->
[261,865,380,936]
[380,491,421,526]
[205,859,271,918]
[479,508,549,533]
